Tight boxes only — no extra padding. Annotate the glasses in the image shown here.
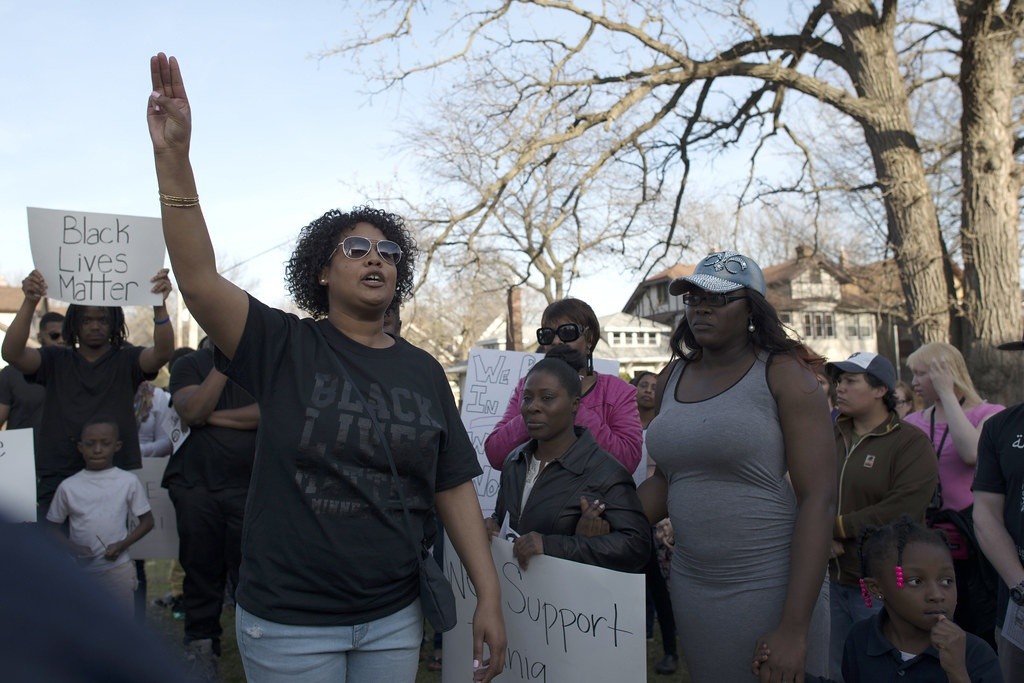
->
[536,323,583,345]
[683,293,748,307]
[327,236,405,266]
[40,331,62,340]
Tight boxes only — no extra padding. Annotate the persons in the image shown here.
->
[46,415,155,617]
[904,342,1006,648]
[132,335,240,620]
[751,516,1004,683]
[817,368,839,421]
[629,372,678,673]
[161,339,260,683]
[484,298,643,476]
[970,403,1024,683]
[427,523,444,670]
[577,253,837,683]
[146,52,506,683]
[2,269,175,627]
[828,351,939,683]
[0,312,66,451]
[894,381,915,419]
[484,358,651,573]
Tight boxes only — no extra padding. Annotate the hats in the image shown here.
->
[824,352,896,391]
[668,251,766,297]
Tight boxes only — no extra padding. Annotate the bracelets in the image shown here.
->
[153,316,169,324]
[159,192,198,207]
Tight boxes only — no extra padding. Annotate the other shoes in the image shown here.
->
[187,638,218,683]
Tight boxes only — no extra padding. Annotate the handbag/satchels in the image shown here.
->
[938,522,969,562]
[417,548,458,631]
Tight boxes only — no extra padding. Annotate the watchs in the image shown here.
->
[1011,581,1024,606]
